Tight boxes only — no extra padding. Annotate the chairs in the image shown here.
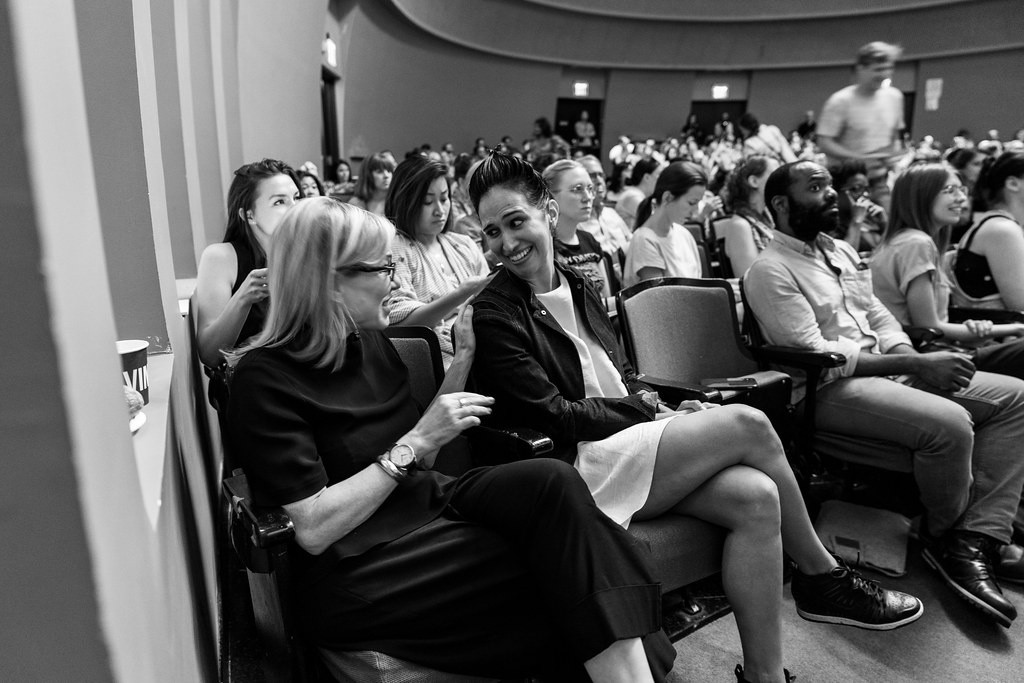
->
[220,224,1024,683]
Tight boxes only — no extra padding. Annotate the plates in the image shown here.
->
[130,410,146,432]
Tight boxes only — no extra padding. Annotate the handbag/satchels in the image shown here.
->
[814,499,911,578]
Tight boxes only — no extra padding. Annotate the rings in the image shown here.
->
[459,399,465,408]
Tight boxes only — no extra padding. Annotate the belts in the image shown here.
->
[868,173,888,186]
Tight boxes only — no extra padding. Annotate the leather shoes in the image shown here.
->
[921,532,1018,629]
[978,538,1024,584]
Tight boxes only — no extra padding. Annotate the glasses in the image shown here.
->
[335,262,396,281]
[939,184,970,199]
[551,185,594,196]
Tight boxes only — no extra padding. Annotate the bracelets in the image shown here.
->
[377,455,405,483]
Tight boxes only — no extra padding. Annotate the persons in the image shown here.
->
[190,129,1024,683]
[714,113,734,140]
[574,111,595,146]
[736,112,799,164]
[797,110,816,136]
[533,117,570,148]
[681,115,703,143]
[816,40,907,215]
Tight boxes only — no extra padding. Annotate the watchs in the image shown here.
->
[386,443,419,476]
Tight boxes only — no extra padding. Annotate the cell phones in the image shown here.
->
[699,378,758,394]
[487,262,504,277]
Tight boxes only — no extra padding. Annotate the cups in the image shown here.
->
[116,340,150,407]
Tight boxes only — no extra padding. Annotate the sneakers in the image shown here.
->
[790,551,924,631]
[735,664,797,683]
